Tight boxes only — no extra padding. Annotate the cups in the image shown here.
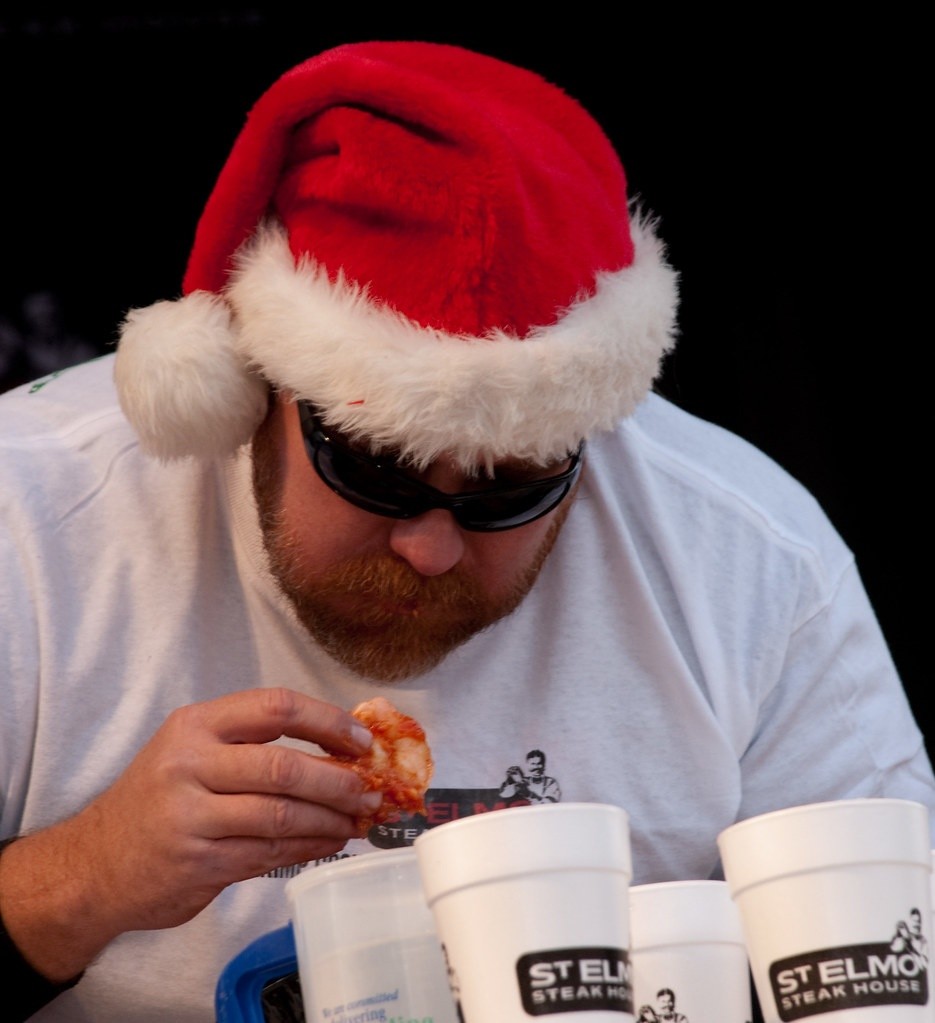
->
[414,801,635,1023]
[717,798,935,1023]
[629,880,752,1023]
[282,845,460,1023]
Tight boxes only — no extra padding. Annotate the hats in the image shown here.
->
[113,41,679,467]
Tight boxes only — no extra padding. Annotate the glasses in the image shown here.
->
[296,392,585,533]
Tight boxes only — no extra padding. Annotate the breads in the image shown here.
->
[312,696,433,837]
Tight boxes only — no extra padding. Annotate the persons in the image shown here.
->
[0,38,935,1023]
